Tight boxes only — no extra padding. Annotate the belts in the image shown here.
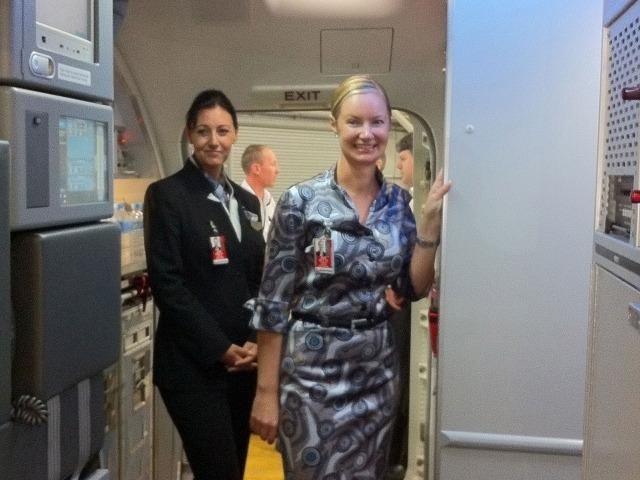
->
[293,307,393,331]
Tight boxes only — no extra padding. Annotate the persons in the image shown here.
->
[238,142,278,241]
[395,131,413,212]
[141,85,266,480]
[316,241,326,256]
[212,238,220,250]
[248,73,448,480]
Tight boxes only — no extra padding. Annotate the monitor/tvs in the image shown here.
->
[36,0,95,63]
[58,114,110,205]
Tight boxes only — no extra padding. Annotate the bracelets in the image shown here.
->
[414,232,442,251]
